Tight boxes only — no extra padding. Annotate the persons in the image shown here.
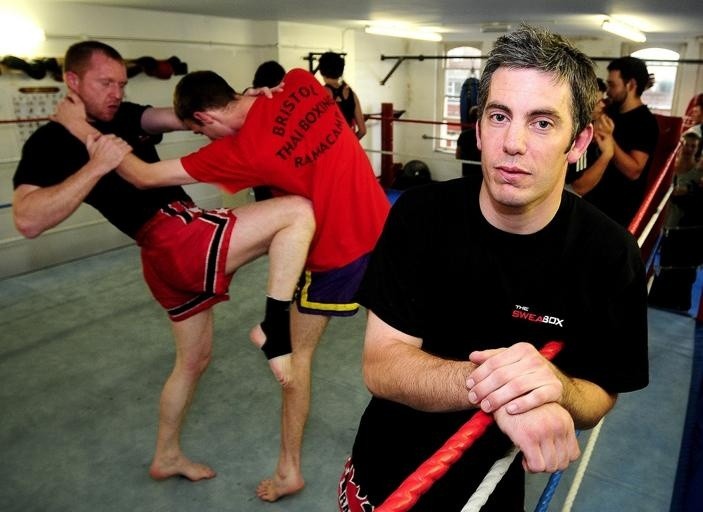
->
[582,55,655,229]
[335,26,649,512]
[10,41,318,483]
[648,93,702,318]
[314,52,365,145]
[48,66,392,500]
[244,61,290,203]
[566,77,616,200]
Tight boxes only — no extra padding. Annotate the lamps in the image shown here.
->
[362,22,442,43]
[599,19,646,43]
